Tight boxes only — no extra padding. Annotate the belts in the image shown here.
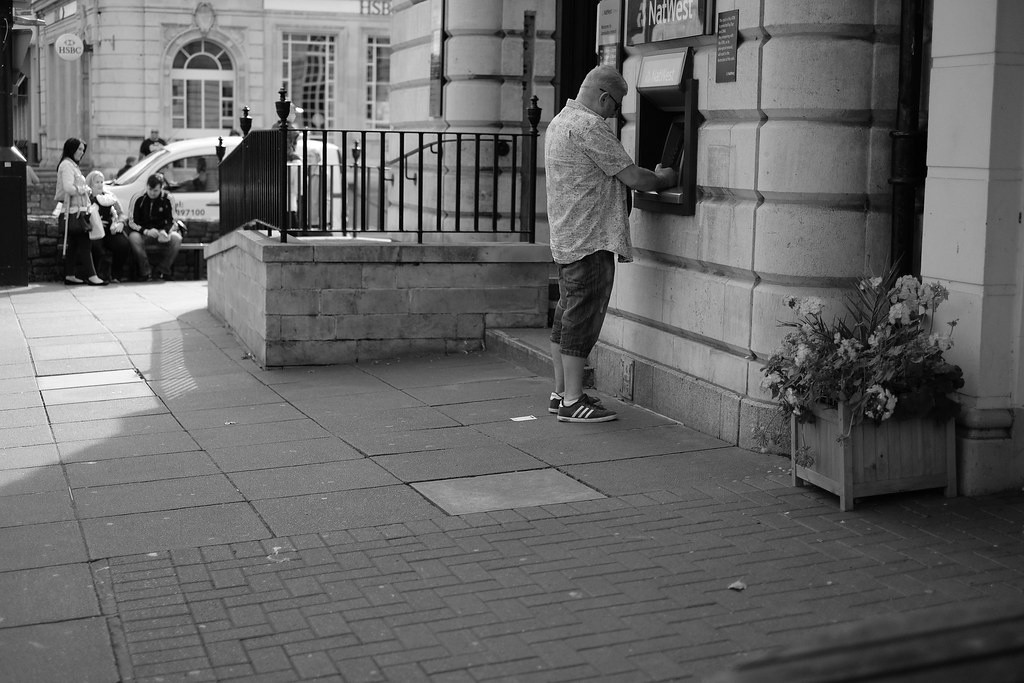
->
[60,206,91,214]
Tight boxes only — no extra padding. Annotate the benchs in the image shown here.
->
[55,242,208,280]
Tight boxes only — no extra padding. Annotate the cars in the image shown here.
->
[53,133,349,250]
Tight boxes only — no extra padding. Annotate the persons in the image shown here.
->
[301,148,334,229]
[138,129,168,165]
[543,64,680,422]
[170,157,206,193]
[81,170,126,284]
[115,156,137,180]
[128,172,183,283]
[26,165,40,186]
[270,102,304,234]
[52,137,106,286]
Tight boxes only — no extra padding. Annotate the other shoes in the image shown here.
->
[64,276,87,285]
[156,271,174,281]
[86,278,109,286]
[144,274,154,282]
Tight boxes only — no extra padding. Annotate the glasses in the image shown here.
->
[600,88,621,112]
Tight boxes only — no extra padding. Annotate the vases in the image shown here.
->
[790,400,959,513]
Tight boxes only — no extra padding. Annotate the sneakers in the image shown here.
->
[548,390,602,414]
[557,393,618,422]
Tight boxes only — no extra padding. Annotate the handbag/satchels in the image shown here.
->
[87,204,106,240]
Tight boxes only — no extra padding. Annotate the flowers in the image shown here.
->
[751,250,964,470]
